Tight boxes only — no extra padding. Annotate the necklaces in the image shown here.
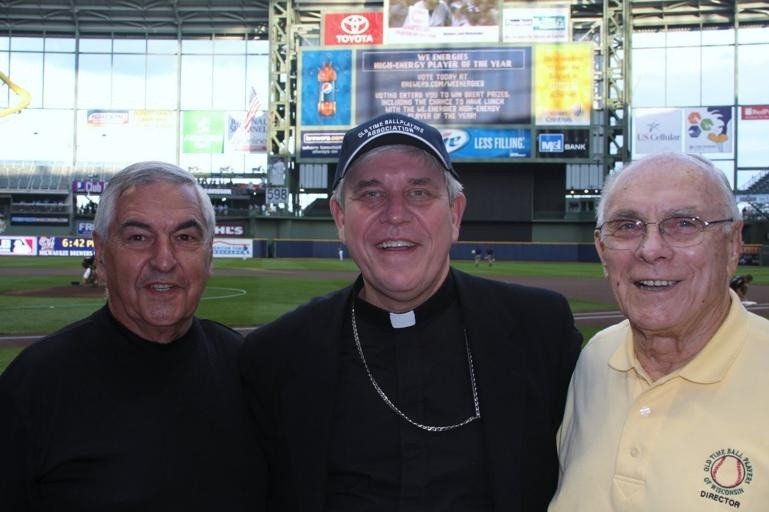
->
[351,289,481,432]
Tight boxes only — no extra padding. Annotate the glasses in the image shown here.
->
[597,216,734,251]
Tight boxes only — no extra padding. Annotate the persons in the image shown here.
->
[730,271,751,300]
[545,151,769,512]
[484,247,496,267]
[471,246,483,268]
[338,245,346,259]
[0,160,244,512]
[244,113,583,512]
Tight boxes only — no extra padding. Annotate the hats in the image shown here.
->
[331,112,462,191]
